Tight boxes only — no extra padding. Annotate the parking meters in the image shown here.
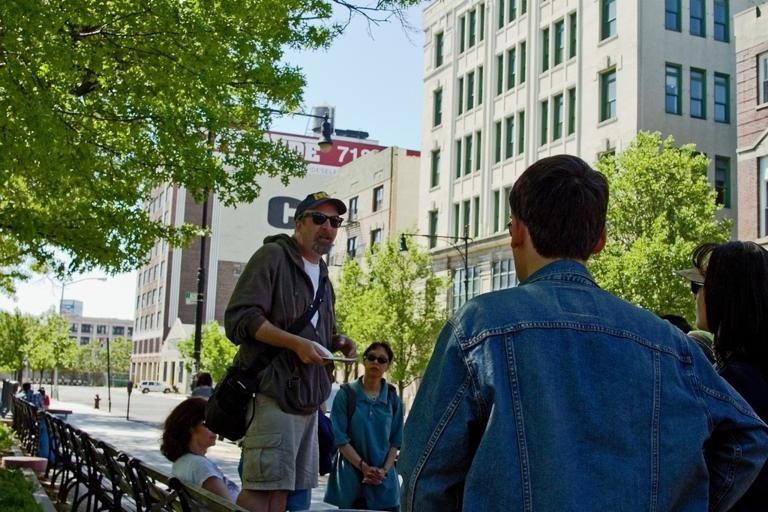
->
[126,378,133,422]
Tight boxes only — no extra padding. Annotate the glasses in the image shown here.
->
[691,281,705,294]
[366,354,388,364]
[310,213,343,228]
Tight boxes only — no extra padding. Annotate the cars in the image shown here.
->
[187,384,217,410]
[322,382,405,464]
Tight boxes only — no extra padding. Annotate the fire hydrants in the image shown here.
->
[90,393,103,412]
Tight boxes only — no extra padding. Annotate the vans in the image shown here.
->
[138,381,171,395]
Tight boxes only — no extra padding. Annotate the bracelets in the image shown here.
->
[359,458,364,473]
[382,465,389,479]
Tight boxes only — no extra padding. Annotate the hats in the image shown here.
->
[675,250,713,284]
[295,191,346,219]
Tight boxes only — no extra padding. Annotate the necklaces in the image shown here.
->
[364,386,380,403]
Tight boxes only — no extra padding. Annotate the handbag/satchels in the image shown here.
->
[204,365,259,440]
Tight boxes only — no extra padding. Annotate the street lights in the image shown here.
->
[397,231,471,316]
[191,94,336,411]
[54,277,111,401]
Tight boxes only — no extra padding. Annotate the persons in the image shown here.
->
[397,153,768,512]
[156,393,242,510]
[321,339,406,512]
[190,374,216,397]
[37,385,52,409]
[222,192,363,511]
[662,311,717,373]
[21,381,44,413]
[673,239,768,512]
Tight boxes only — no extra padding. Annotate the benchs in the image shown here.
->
[2,378,250,512]
